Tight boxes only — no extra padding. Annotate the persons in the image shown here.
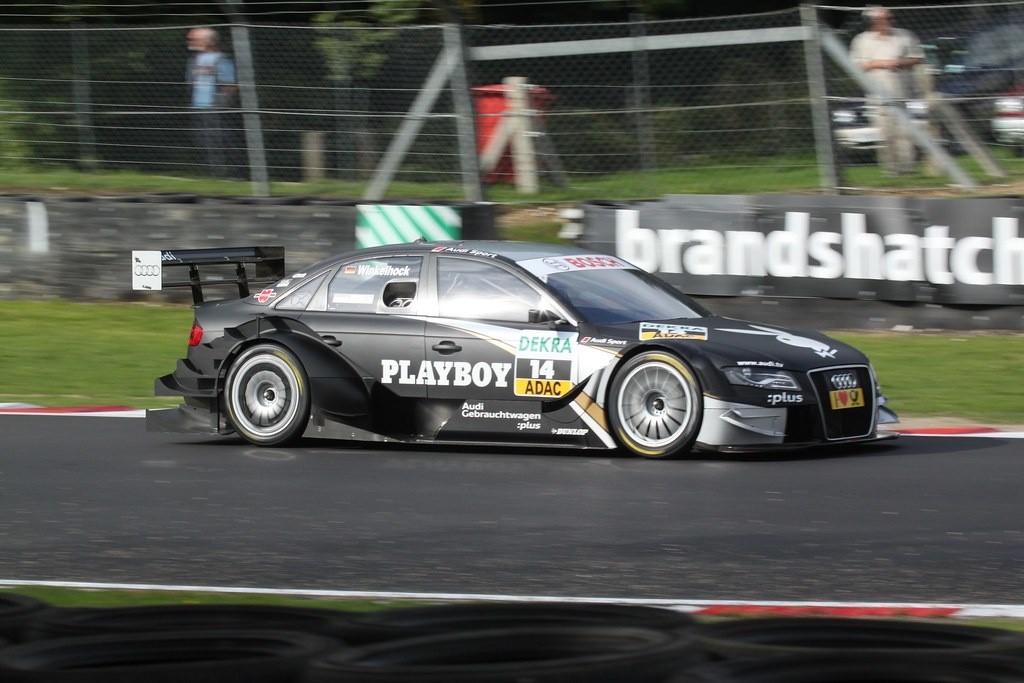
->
[183,27,241,177]
[849,5,927,181]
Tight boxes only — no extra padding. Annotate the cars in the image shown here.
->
[132,240,902,465]
[821,34,1024,165]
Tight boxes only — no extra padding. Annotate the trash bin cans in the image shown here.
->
[472,82,555,179]
